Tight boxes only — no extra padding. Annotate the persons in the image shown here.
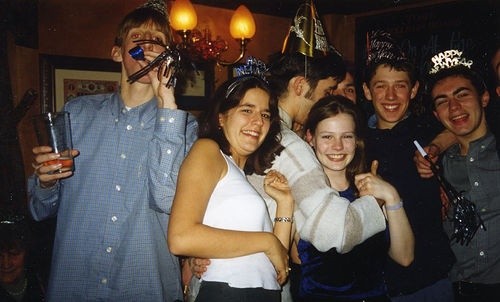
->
[167,55,295,302]
[27,0,500,302]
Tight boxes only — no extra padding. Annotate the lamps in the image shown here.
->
[167,0,257,66]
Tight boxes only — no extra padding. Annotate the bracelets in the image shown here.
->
[274,217,293,223]
[386,199,404,211]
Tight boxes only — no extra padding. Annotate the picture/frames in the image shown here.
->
[39,54,122,114]
[174,63,260,112]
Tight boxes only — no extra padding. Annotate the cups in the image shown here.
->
[32,111,75,175]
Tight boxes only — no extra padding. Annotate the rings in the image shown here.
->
[286,267,292,272]
